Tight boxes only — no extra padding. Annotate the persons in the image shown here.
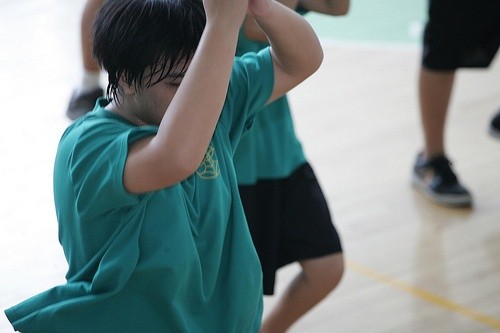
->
[63,0,106,123]
[410,0,499,207]
[5,0,324,333]
[224,0,354,333]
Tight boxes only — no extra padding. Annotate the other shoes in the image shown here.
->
[66,88,104,121]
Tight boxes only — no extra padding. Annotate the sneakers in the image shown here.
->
[412,151,470,206]
